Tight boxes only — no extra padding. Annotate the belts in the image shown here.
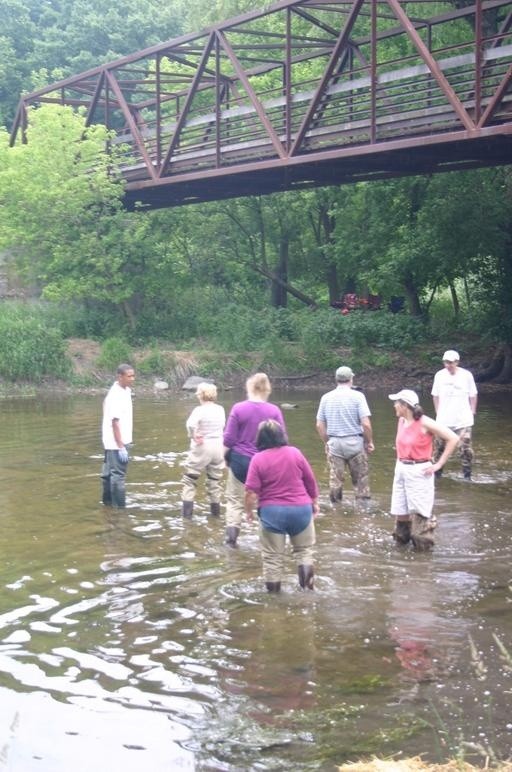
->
[403,460,428,464]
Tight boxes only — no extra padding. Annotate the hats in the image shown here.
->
[388,389,419,407]
[335,365,355,381]
[442,349,460,362]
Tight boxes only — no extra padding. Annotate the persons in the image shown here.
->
[244,418,321,596]
[432,349,478,481]
[314,366,375,506]
[100,363,137,509]
[180,382,227,518]
[388,389,460,556]
[222,372,289,546]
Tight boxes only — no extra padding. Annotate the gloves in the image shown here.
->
[118,447,129,463]
